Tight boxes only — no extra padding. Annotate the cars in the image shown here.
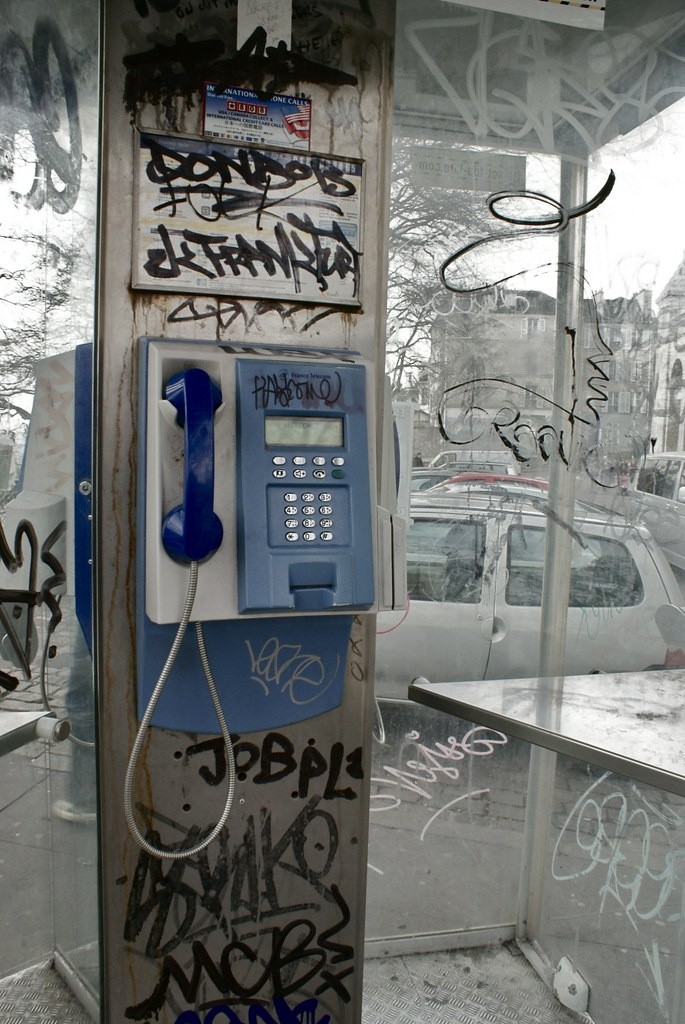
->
[625,449,685,573]
[368,449,685,696]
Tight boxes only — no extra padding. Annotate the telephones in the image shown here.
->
[146,341,408,626]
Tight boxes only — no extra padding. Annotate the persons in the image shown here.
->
[589,455,637,496]
[412,452,424,467]
